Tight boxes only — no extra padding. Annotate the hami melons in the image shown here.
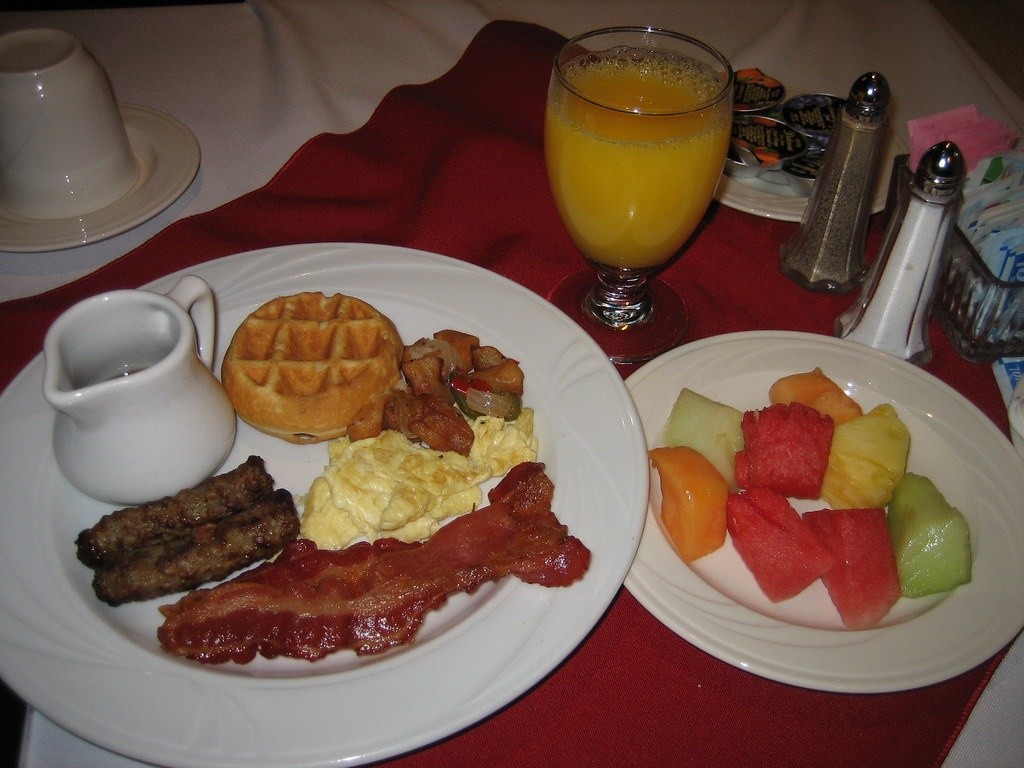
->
[769,368,863,426]
[647,446,729,562]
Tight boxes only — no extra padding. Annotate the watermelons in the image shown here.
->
[724,403,901,631]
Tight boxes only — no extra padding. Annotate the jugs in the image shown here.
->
[41,273,238,506]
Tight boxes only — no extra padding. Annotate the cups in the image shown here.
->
[1,29,140,221]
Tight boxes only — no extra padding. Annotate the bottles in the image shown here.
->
[778,71,894,293]
[834,137,968,366]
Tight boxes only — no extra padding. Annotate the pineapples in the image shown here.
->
[820,405,910,508]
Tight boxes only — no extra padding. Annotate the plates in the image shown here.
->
[1,103,202,253]
[716,88,910,222]
[1,240,650,767]
[624,331,1022,697]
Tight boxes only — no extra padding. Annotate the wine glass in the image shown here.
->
[539,26,735,361]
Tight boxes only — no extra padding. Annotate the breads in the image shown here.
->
[220,290,402,442]
